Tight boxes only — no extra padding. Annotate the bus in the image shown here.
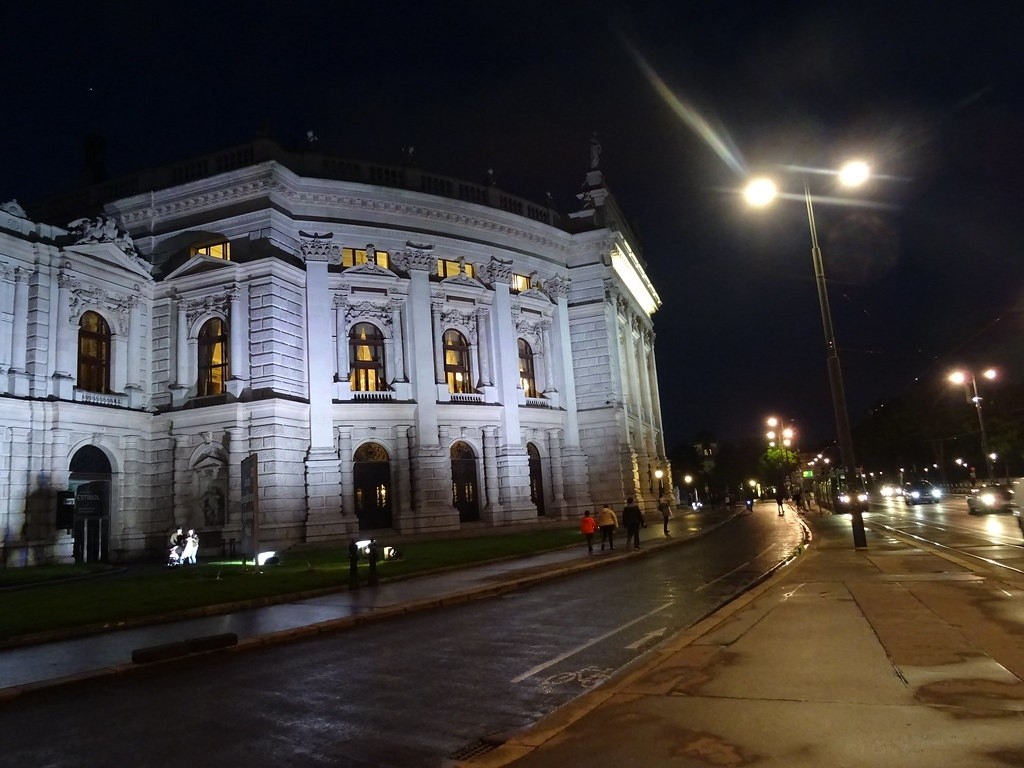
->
[830,471,870,515]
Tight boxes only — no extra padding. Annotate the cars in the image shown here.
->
[902,479,941,507]
[964,483,1016,515]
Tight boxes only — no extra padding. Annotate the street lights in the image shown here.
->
[947,366,998,485]
[744,158,876,550]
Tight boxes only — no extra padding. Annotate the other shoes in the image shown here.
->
[589,546,593,551]
[602,544,604,551]
[611,549,615,550]
[627,539,631,544]
[665,530,670,534]
[635,546,641,549]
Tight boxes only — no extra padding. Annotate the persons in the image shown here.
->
[198,489,223,527]
[166,527,199,568]
[579,511,597,553]
[621,497,647,550]
[596,504,620,551]
[687,488,813,513]
[658,494,671,533]
